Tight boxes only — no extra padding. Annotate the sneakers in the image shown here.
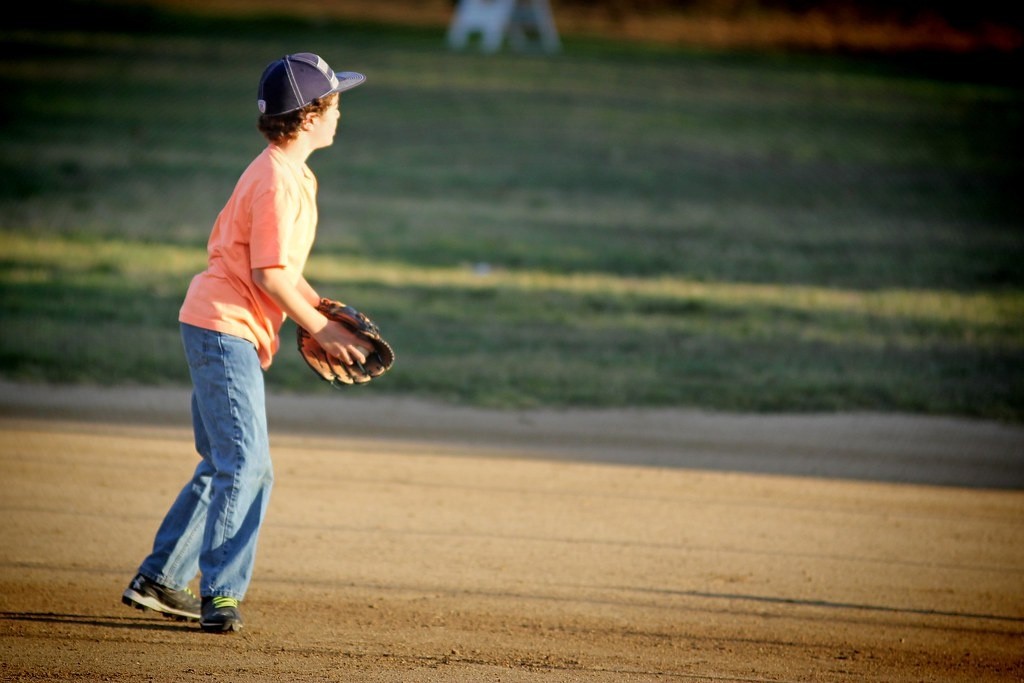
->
[199,597,244,633]
[121,573,201,623]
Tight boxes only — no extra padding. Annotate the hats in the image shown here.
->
[256,51,366,117]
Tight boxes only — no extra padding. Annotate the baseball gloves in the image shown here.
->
[298,297,395,388]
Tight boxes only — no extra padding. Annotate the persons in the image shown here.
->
[120,53,375,630]
